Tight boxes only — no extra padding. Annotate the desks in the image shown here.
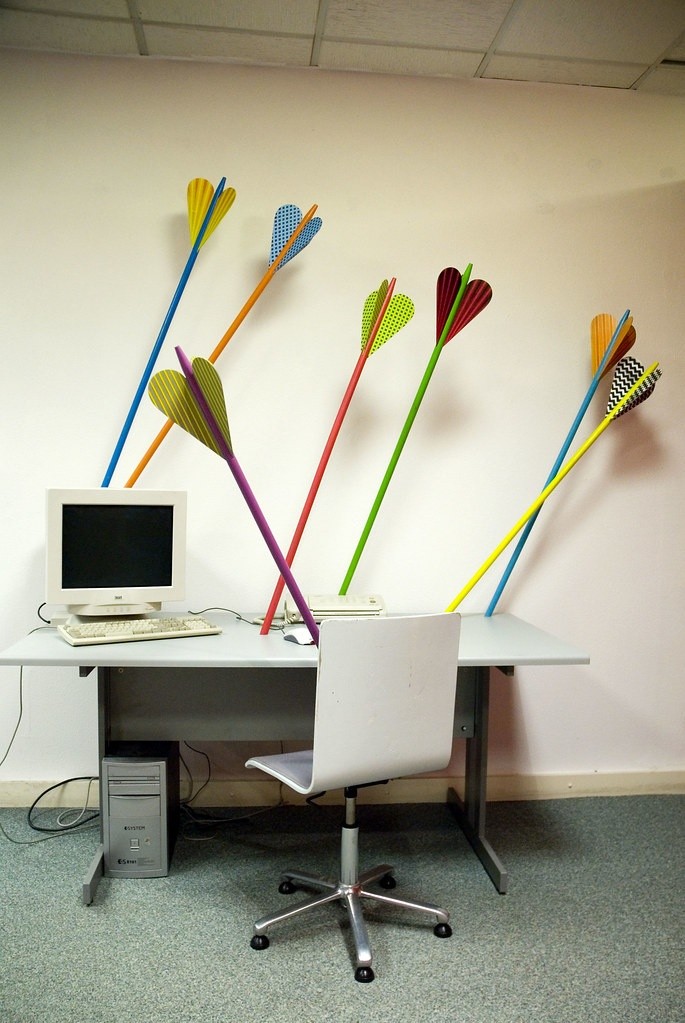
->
[0,601,596,914]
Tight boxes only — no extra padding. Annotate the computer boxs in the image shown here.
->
[102,740,180,877]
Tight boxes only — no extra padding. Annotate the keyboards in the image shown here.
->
[56,617,223,646]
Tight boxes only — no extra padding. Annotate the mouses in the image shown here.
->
[283,628,317,646]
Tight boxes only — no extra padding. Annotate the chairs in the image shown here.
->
[241,610,467,991]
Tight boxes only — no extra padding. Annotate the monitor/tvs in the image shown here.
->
[44,488,187,617]
